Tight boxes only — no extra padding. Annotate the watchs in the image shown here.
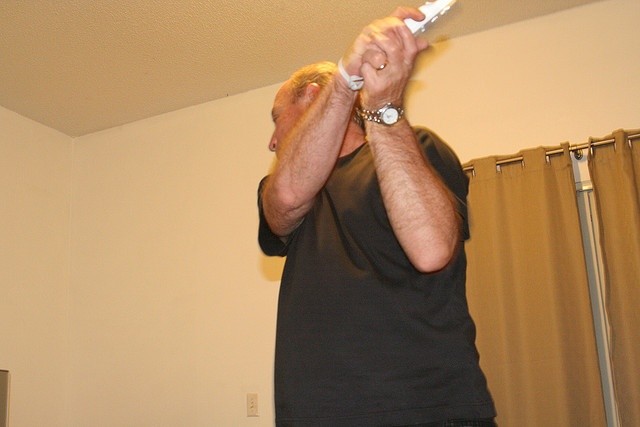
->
[351,102,404,126]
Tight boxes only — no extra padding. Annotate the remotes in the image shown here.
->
[402,0,461,37]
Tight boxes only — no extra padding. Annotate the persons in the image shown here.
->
[257,4,498,427]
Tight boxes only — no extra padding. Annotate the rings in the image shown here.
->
[375,63,387,70]
